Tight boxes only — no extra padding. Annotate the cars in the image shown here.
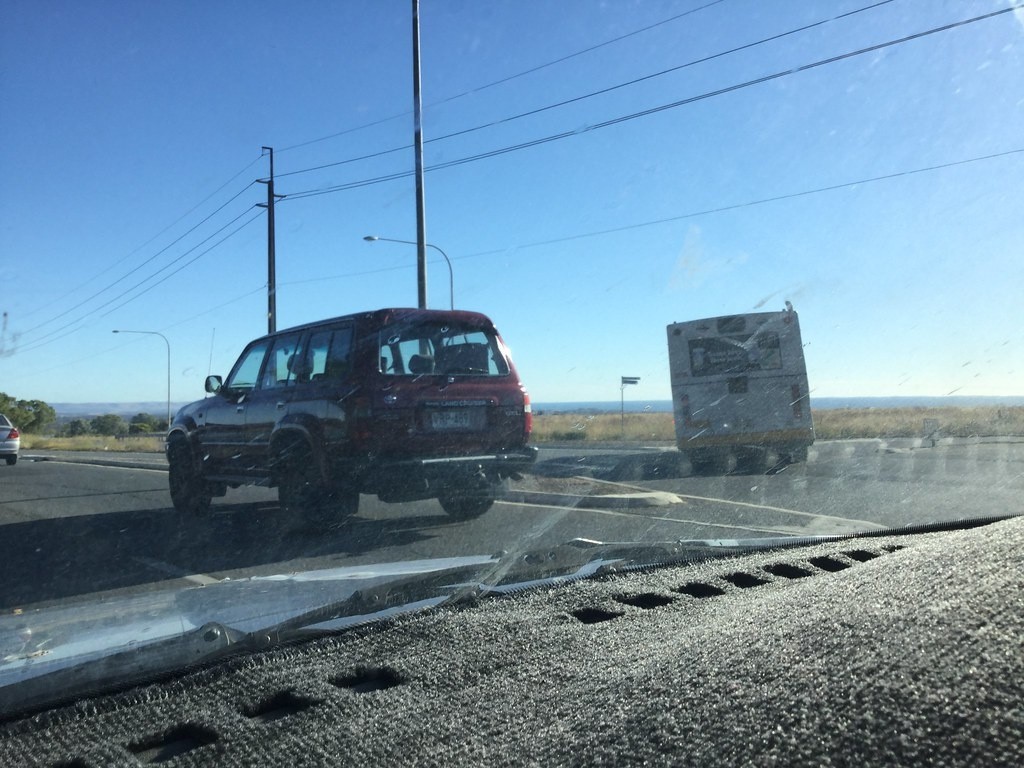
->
[0,413,20,466]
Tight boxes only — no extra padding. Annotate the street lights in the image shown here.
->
[364,235,454,311]
[112,330,173,430]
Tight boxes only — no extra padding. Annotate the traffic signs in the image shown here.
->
[622,381,638,385]
[622,377,640,381]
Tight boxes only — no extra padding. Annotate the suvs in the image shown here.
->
[162,308,539,536]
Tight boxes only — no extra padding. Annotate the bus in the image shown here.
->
[666,300,817,473]
